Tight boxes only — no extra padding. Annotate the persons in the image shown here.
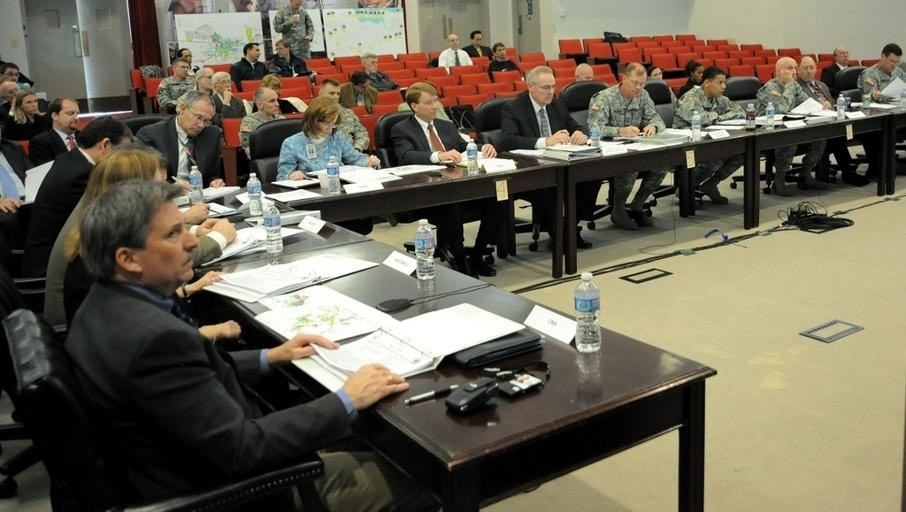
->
[58,177,443,512]
[821,47,853,86]
[857,44,906,185]
[1,39,381,342]
[387,81,506,281]
[679,59,705,97]
[753,55,869,196]
[397,83,454,122]
[673,67,746,208]
[500,66,602,249]
[274,0,315,61]
[487,41,521,82]
[587,62,667,231]
[462,30,494,60]
[437,33,474,68]
[168,0,203,21]
[575,63,594,83]
[647,65,678,104]
[361,52,400,93]
[358,0,399,8]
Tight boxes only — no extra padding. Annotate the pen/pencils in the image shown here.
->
[404,384,459,406]
[210,208,221,215]
[171,176,178,181]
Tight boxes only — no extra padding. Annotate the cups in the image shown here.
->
[846,97,851,110]
[863,94,872,109]
[318,173,328,187]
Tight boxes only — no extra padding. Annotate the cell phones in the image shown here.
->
[445,376,500,413]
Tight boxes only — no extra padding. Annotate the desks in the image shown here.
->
[850,98,905,195]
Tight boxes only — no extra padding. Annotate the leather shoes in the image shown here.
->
[453,254,495,279]
[841,173,870,187]
[577,232,592,249]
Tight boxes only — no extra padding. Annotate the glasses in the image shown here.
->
[188,109,211,127]
[320,120,337,129]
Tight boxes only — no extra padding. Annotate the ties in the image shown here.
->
[454,51,460,66]
[538,109,550,138]
[65,134,76,150]
[428,125,445,152]
[187,140,196,172]
[811,83,824,100]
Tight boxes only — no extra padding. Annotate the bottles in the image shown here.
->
[466,138,478,174]
[901,88,906,110]
[189,165,205,205]
[572,272,602,355]
[414,217,437,280]
[746,103,757,130]
[590,123,600,146]
[262,200,283,254]
[246,172,263,217]
[692,110,702,140]
[837,94,845,120]
[766,102,775,129]
[326,156,342,194]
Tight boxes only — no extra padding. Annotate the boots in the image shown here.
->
[701,171,729,204]
[610,198,638,231]
[675,176,700,209]
[629,190,652,225]
[772,171,792,196]
[798,166,827,190]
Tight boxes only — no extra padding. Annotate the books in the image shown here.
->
[432,301,548,365]
